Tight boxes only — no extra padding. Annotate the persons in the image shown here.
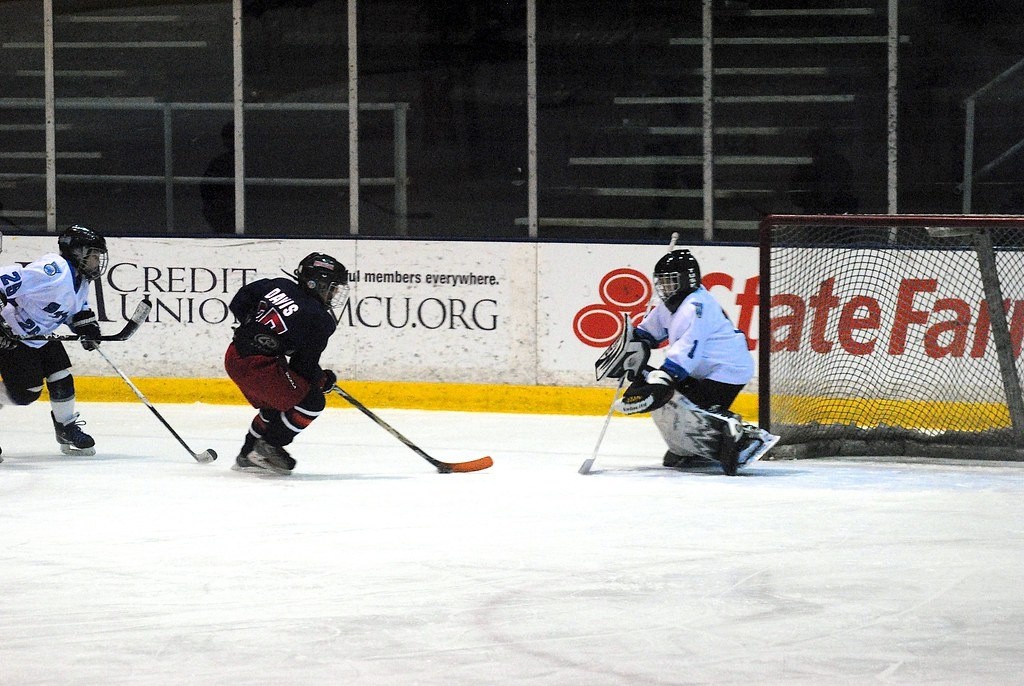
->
[0,223,111,450]
[222,252,349,473]
[608,250,772,476]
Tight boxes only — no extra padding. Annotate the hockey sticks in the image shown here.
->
[11,298,153,341]
[332,385,493,474]
[577,377,626,474]
[91,342,219,464]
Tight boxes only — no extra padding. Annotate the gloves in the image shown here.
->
[321,370,336,395]
[0,314,18,351]
[72,310,101,351]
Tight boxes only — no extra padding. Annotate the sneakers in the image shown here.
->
[731,429,780,469]
[233,443,257,472]
[51,410,96,456]
[247,438,296,476]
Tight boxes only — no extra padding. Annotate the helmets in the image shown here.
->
[297,252,348,311]
[655,249,699,312]
[58,225,107,282]
